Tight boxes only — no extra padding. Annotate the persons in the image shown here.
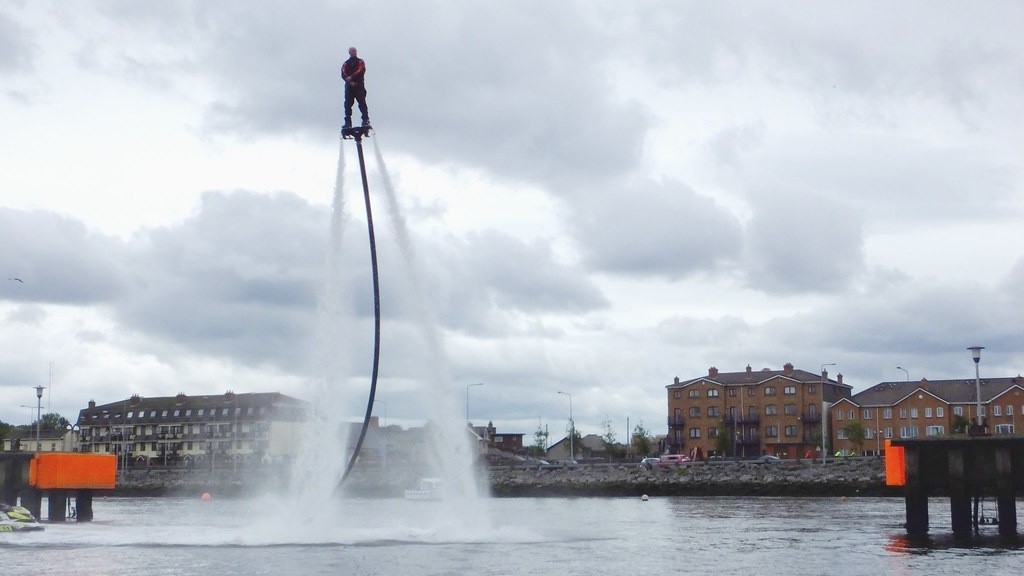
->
[805,450,814,460]
[341,46,371,129]
[835,450,841,456]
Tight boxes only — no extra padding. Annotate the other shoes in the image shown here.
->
[342,121,353,128]
[361,119,370,126]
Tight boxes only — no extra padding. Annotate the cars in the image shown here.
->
[758,454,780,461]
[641,458,660,464]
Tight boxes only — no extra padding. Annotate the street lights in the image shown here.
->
[372,399,387,469]
[967,345,987,425]
[466,382,485,426]
[558,390,574,460]
[820,362,837,402]
[897,366,912,436]
[32,384,47,451]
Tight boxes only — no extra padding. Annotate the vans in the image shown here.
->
[659,454,690,463]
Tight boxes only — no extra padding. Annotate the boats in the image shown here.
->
[405,478,446,499]
